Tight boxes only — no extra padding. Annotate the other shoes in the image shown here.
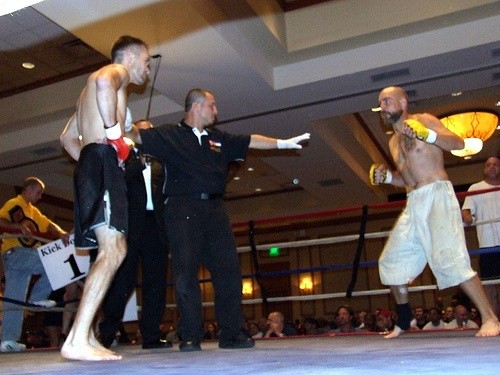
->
[220,333,255,348]
[142,336,174,349]
[100,319,119,349]
[179,339,201,352]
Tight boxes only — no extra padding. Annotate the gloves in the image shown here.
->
[277,132,311,149]
[104,121,134,167]
[125,108,133,132]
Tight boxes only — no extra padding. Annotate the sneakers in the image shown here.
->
[31,299,57,308]
[0,341,27,353]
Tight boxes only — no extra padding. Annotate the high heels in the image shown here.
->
[57,333,66,346]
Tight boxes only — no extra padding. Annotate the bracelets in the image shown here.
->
[425,129,437,144]
[384,169,392,184]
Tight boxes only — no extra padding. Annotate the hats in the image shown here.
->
[377,309,392,318]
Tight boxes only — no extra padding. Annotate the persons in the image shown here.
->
[0,177,68,352]
[378,309,396,331]
[296,305,482,336]
[461,157,500,315]
[125,88,311,352]
[132,327,142,344]
[97,119,173,349]
[60,34,150,361]
[160,322,178,341]
[329,306,367,332]
[206,322,217,339]
[369,86,500,339]
[245,318,266,335]
[263,312,298,338]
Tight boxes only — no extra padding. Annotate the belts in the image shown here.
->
[168,192,217,200]
